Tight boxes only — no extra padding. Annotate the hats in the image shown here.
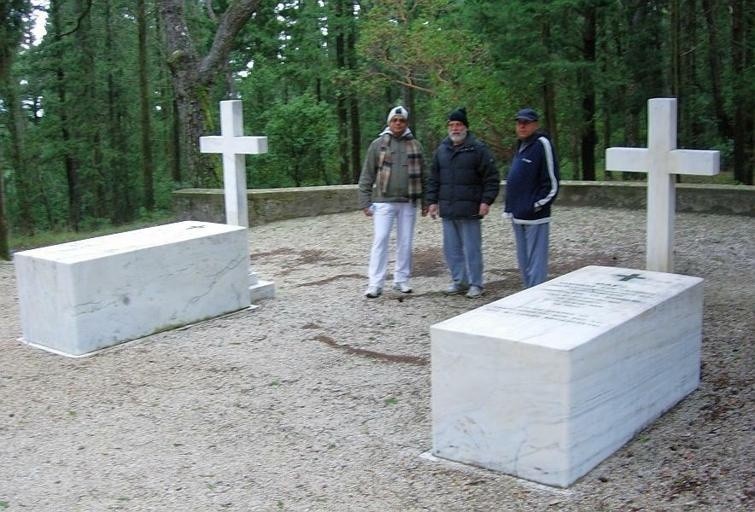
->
[449,107,469,127]
[515,108,538,121]
[387,106,408,126]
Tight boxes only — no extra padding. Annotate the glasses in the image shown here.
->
[391,118,406,123]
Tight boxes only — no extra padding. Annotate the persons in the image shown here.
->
[505,108,560,288]
[427,106,500,299]
[358,106,429,297]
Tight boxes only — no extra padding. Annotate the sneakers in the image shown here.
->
[447,286,469,295]
[366,286,382,298]
[466,286,486,298]
[393,282,411,293]
